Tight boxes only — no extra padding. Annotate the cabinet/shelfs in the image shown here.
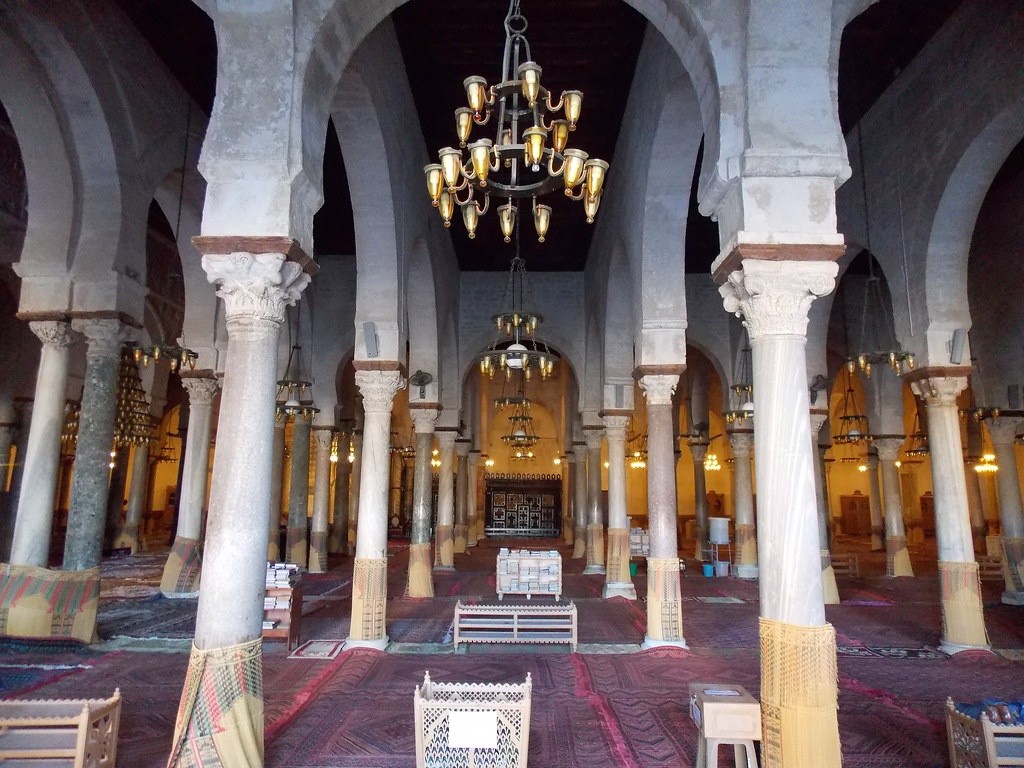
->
[496,548,562,600]
[830,554,859,579]
[454,600,577,653]
[840,490,870,534]
[0,688,122,768]
[919,491,935,536]
[943,696,1024,768]
[262,562,305,651]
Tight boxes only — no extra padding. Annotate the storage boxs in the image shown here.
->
[689,684,763,740]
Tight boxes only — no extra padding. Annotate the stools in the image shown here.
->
[696,731,758,768]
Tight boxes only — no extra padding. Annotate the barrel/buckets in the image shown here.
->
[708,517,731,542]
[715,561,730,577]
[703,564,714,577]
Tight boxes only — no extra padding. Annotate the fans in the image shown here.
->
[408,370,433,399]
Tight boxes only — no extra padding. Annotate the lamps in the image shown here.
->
[624,414,649,468]
[59,342,161,448]
[724,447,734,470]
[151,410,179,464]
[389,411,404,453]
[904,410,929,457]
[400,424,416,458]
[495,366,540,461]
[276,300,322,424]
[477,170,561,381]
[957,358,1000,423]
[425,0,610,244]
[132,324,199,373]
[848,95,917,378]
[840,446,860,463]
[833,371,874,446]
[725,328,753,425]
